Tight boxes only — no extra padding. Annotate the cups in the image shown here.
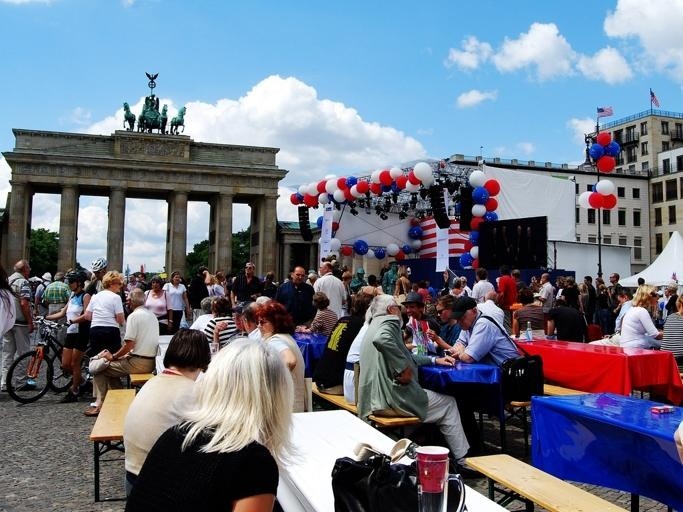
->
[413,444,448,493]
[416,460,465,511]
[209,342,218,354]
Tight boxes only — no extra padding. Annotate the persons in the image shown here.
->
[122,327,211,497]
[1,257,535,414]
[144,93,159,117]
[531,271,682,374]
[119,336,295,511]
[359,292,482,474]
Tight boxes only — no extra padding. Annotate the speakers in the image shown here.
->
[460,189,472,231]
[429,187,451,229]
[298,206,313,241]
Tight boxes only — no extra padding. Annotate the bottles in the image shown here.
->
[415,324,427,356]
[525,321,532,341]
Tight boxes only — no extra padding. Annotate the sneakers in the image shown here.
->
[76,381,94,398]
[17,381,38,392]
[84,404,103,417]
[1,384,16,393]
[64,386,81,403]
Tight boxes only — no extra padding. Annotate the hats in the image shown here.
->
[401,292,426,306]
[448,296,477,320]
[41,272,52,282]
[151,275,162,283]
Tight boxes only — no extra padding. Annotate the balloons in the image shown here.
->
[288,161,499,271]
[578,178,616,211]
[595,131,610,145]
[595,155,615,173]
[600,140,620,156]
[587,143,604,160]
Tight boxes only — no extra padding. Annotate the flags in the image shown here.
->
[596,105,615,118]
[649,88,660,109]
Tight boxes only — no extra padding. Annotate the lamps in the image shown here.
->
[334,188,432,221]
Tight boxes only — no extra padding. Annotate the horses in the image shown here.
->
[122,101,135,132]
[170,107,186,135]
[160,104,167,135]
[137,104,147,133]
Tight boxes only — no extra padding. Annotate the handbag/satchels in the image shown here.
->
[331,436,464,512]
[502,355,545,403]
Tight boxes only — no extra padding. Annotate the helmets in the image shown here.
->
[65,267,87,282]
[90,257,107,272]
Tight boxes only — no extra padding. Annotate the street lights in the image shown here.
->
[583,134,602,284]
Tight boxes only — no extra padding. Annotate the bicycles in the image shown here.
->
[5,314,91,404]
